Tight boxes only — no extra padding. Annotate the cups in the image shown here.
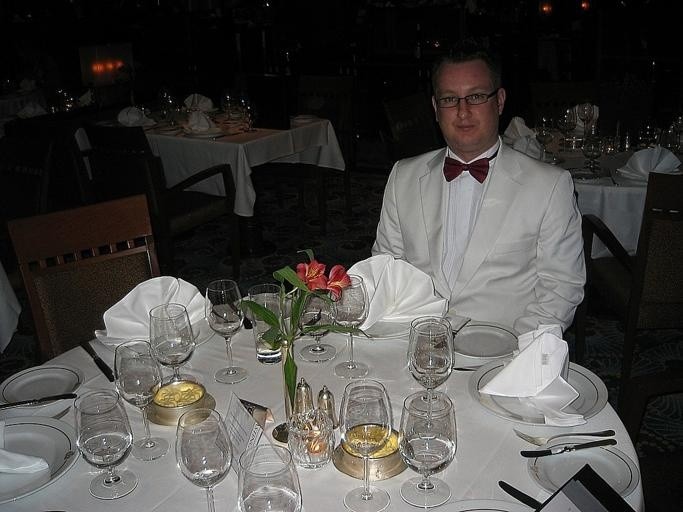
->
[236,445,302,512]
[288,413,333,469]
[248,284,283,364]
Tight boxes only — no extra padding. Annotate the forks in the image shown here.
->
[512,428,618,446]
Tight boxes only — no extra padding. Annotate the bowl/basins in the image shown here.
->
[153,380,206,419]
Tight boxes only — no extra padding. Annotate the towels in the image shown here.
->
[19,76,37,91]
[565,103,600,128]
[616,143,682,181]
[502,115,545,160]
[0,420,50,475]
[76,88,93,108]
[183,92,214,113]
[17,100,48,119]
[330,254,447,332]
[477,323,587,428]
[186,111,217,133]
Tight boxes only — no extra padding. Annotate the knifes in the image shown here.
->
[453,364,481,373]
[0,389,77,412]
[605,168,619,186]
[435,316,472,349]
[497,478,546,509]
[520,439,617,458]
[51,406,71,419]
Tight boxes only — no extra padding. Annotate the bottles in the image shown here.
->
[338,380,396,511]
[291,376,313,412]
[315,386,338,431]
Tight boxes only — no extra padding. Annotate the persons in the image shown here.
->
[370,33,588,337]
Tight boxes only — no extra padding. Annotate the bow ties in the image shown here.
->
[443,156,489,184]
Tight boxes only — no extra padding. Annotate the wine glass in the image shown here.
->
[402,317,457,439]
[148,303,196,384]
[396,393,457,508]
[135,89,179,130]
[533,99,682,173]
[175,408,234,512]
[329,272,371,377]
[293,282,338,363]
[220,93,256,134]
[113,339,170,462]
[204,279,248,384]
[74,388,138,501]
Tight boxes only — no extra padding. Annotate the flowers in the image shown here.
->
[239,247,352,414]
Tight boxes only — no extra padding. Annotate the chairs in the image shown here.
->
[618,359,683,510]
[574,171,683,411]
[0,110,242,362]
[275,87,353,217]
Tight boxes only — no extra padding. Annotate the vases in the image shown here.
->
[273,343,298,443]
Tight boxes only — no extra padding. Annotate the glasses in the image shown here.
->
[433,88,499,109]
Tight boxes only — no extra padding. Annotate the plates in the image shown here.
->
[0,364,84,409]
[468,356,609,427]
[144,391,216,426]
[452,321,520,359]
[344,318,417,340]
[0,414,80,503]
[432,499,530,512]
[238,293,299,324]
[527,441,639,497]
[184,127,224,138]
